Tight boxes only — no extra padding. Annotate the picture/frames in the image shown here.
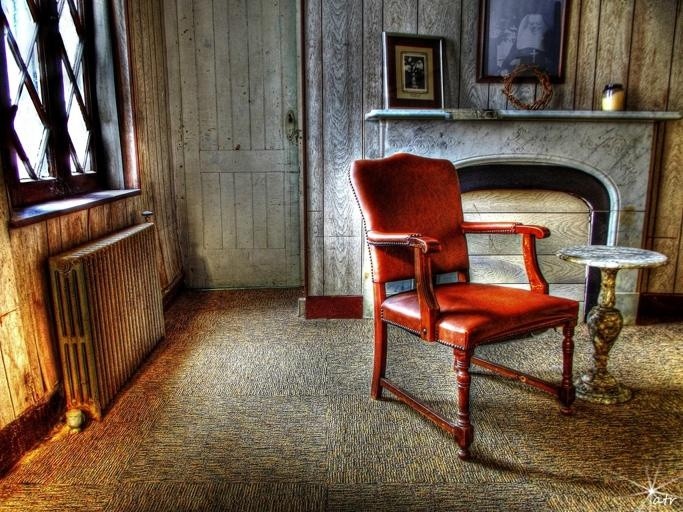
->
[473,0,570,85]
[381,30,445,111]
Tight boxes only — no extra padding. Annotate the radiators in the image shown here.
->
[45,220,166,421]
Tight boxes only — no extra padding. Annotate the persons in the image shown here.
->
[497,11,559,79]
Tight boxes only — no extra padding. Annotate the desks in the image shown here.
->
[551,244,669,406]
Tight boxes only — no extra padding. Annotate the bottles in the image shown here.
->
[600,82,626,112]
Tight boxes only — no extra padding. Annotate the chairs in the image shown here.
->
[348,152,579,458]
